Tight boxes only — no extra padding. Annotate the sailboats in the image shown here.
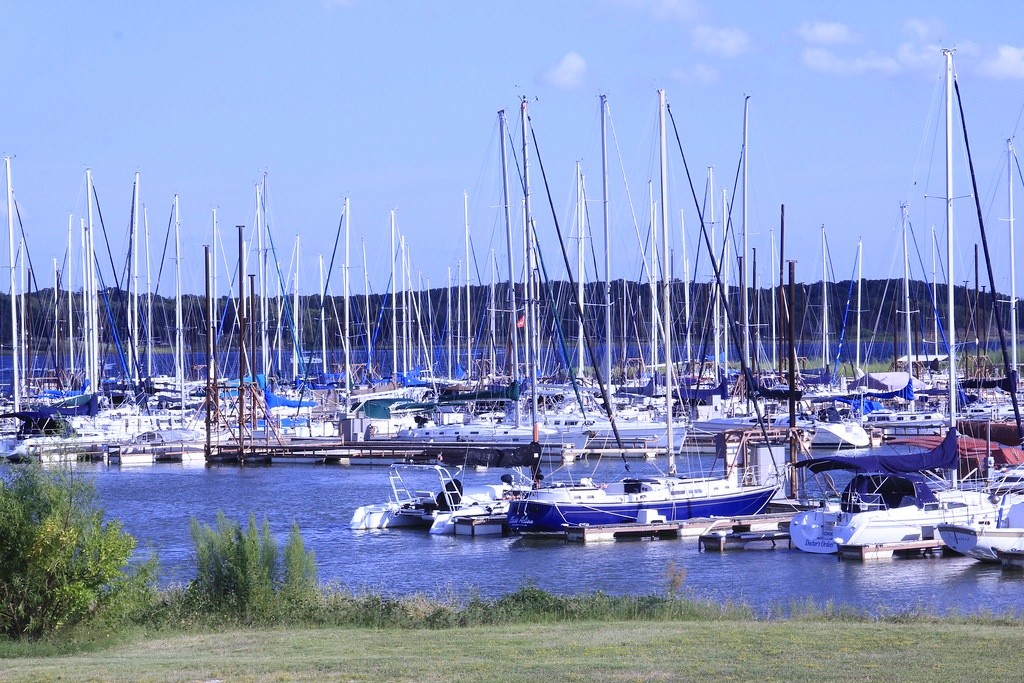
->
[502,87,782,540]
[789,49,1024,557]
[1,93,1024,478]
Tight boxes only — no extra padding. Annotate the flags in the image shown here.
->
[516,314,525,328]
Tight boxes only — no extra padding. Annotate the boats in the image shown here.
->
[937,463,1024,568]
[348,464,531,535]
